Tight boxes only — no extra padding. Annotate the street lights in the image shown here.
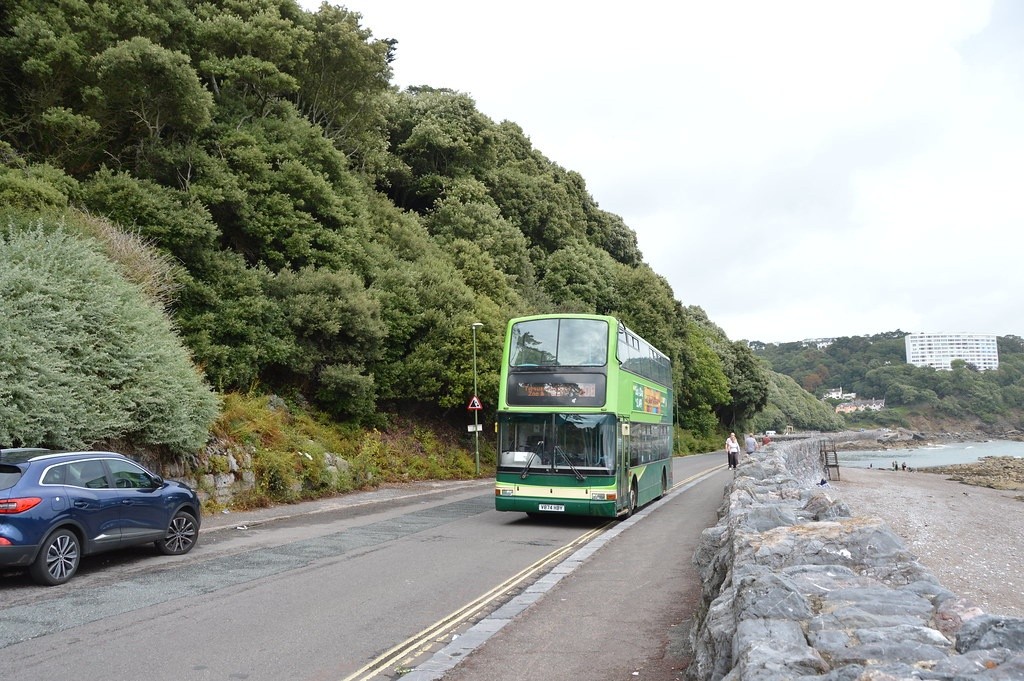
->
[472,323,485,479]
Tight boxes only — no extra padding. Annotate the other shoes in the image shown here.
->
[729,468,731,471]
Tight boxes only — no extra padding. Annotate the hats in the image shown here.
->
[731,433,735,435]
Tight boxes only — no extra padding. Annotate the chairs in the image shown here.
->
[541,353,553,364]
[80,463,105,480]
[526,354,538,364]
[525,437,547,452]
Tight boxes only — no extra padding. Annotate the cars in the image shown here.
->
[0,447,203,586]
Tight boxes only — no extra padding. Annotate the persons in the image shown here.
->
[744,432,758,455]
[762,434,771,446]
[892,461,907,471]
[725,433,740,470]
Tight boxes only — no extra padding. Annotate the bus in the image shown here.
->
[493,314,674,522]
